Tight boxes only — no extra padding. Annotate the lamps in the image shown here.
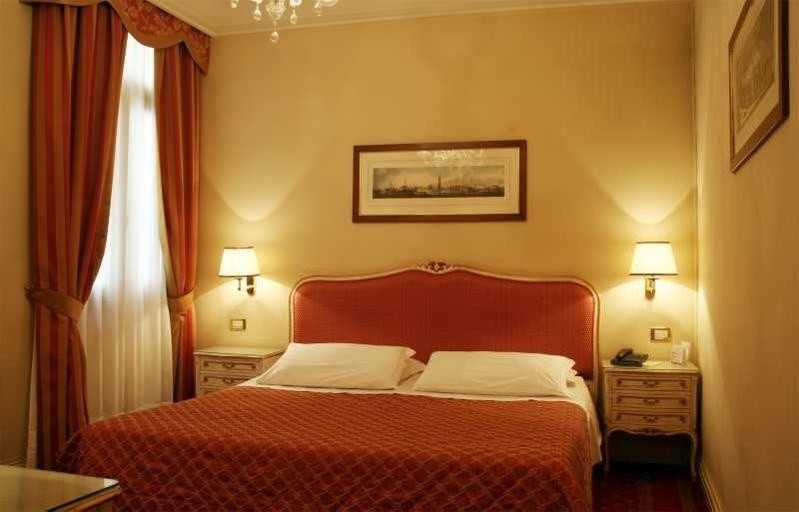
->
[216,246,262,295]
[628,240,679,301]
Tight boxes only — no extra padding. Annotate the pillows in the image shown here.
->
[401,358,426,382]
[566,368,578,384]
[411,351,577,398]
[256,342,416,390]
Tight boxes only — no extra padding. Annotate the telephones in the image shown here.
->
[611,346,649,367]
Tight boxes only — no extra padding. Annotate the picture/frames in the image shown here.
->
[726,0,790,174]
[352,139,527,222]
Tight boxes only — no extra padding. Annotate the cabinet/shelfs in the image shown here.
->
[602,360,699,478]
[192,346,285,396]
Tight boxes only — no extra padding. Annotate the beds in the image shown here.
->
[53,263,599,512]
